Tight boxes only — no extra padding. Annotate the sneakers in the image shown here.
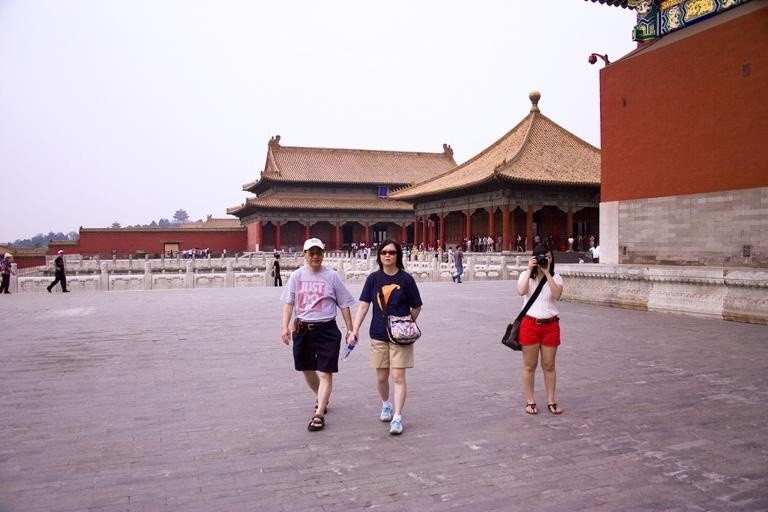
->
[389,415,404,434]
[379,402,394,422]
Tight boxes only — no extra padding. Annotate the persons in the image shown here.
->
[350,241,382,260]
[516,233,600,264]
[280,238,354,431]
[273,254,282,286]
[0,253,16,294]
[47,250,70,293]
[516,244,563,414]
[399,233,502,283]
[170,248,210,259]
[223,248,226,258]
[347,238,423,434]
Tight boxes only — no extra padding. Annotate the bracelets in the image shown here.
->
[347,329,353,335]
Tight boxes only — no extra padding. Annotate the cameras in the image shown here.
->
[536,255,548,266]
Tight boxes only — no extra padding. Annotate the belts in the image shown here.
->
[294,319,336,331]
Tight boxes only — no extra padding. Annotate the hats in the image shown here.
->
[302,237,326,254]
[4,253,12,258]
[58,250,64,254]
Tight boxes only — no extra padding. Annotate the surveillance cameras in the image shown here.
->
[589,56,597,64]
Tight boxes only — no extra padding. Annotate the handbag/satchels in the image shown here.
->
[501,321,523,352]
[384,313,422,346]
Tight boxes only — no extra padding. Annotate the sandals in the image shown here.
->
[313,398,329,415]
[525,401,539,414]
[546,402,562,414]
[308,414,326,432]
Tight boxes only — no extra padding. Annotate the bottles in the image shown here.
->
[338,333,359,362]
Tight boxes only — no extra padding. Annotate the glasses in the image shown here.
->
[378,250,398,256]
